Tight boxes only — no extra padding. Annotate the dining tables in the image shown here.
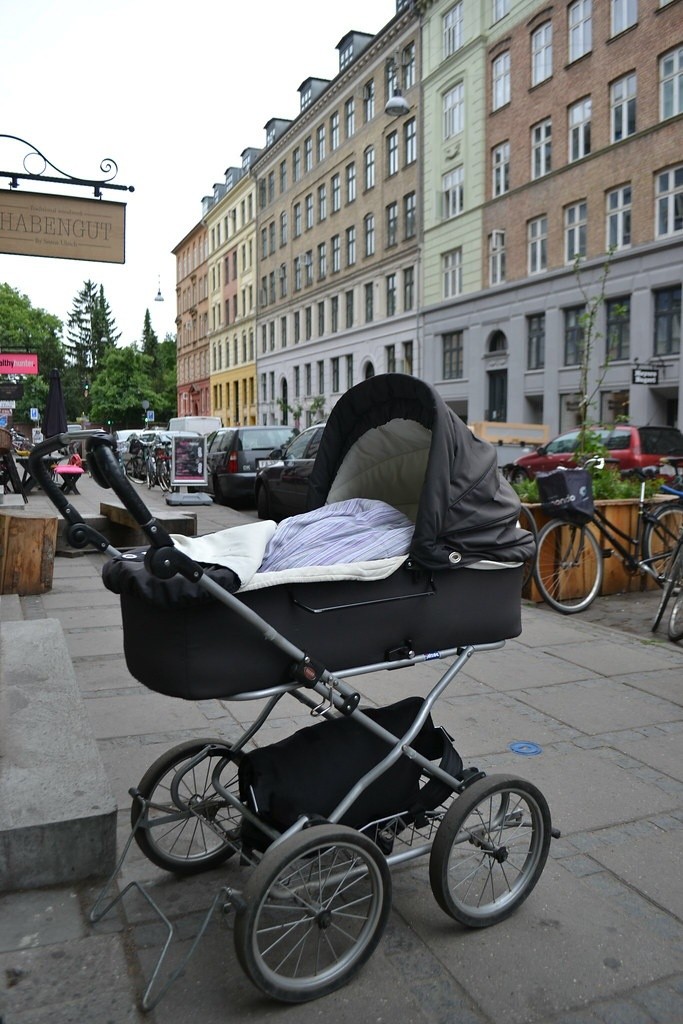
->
[16,455,65,495]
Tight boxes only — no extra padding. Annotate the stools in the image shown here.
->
[53,465,85,495]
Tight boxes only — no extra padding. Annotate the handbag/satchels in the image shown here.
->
[240,698,462,862]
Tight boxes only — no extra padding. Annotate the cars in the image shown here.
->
[58,425,82,456]
[114,429,146,451]
[123,430,199,479]
[254,422,326,522]
[186,426,299,506]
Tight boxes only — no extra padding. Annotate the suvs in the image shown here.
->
[509,425,683,491]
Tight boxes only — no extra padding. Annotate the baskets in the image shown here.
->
[128,439,142,454]
[536,469,593,524]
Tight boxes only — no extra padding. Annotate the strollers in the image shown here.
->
[29,371,560,1015]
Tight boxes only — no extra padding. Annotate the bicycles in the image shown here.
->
[652,535,683,644]
[126,439,179,496]
[536,463,682,615]
[521,504,538,590]
[8,425,35,455]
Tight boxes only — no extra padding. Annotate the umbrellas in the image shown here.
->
[40,365,70,439]
